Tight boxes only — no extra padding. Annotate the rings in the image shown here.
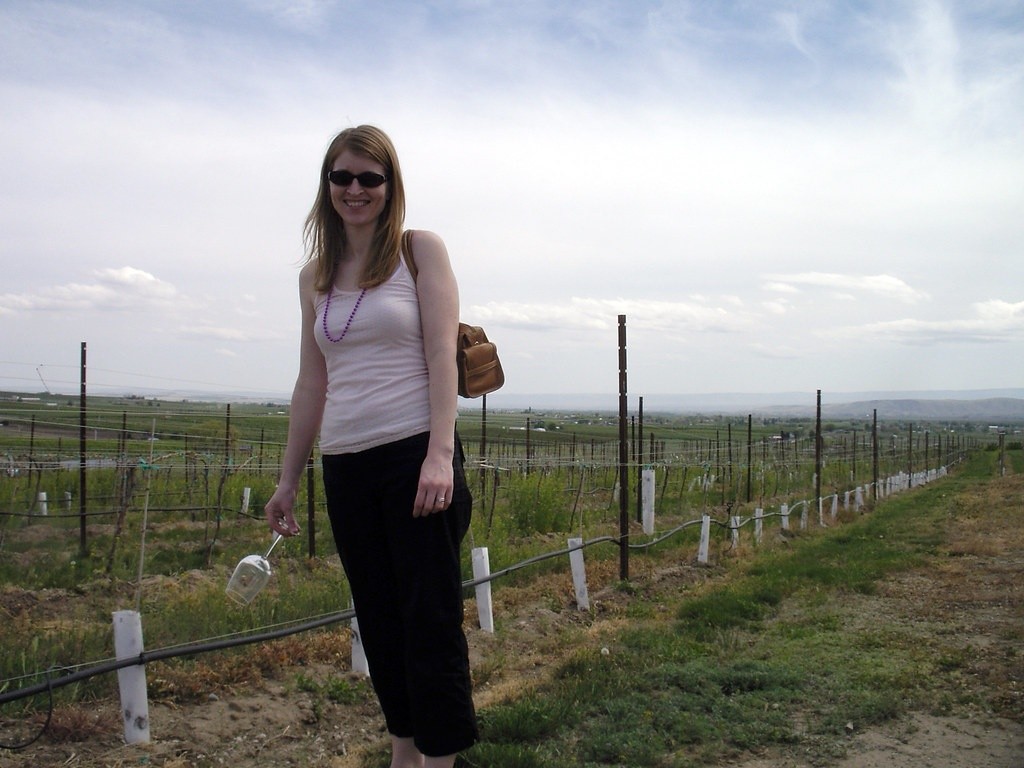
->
[435,497,446,504]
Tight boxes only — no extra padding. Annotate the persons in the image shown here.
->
[264,124,473,767]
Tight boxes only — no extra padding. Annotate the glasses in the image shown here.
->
[327,170,391,188]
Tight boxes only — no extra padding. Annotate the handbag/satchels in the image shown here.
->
[400,229,505,398]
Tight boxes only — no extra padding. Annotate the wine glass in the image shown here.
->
[225,518,299,606]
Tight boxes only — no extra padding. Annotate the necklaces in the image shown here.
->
[322,225,388,343]
